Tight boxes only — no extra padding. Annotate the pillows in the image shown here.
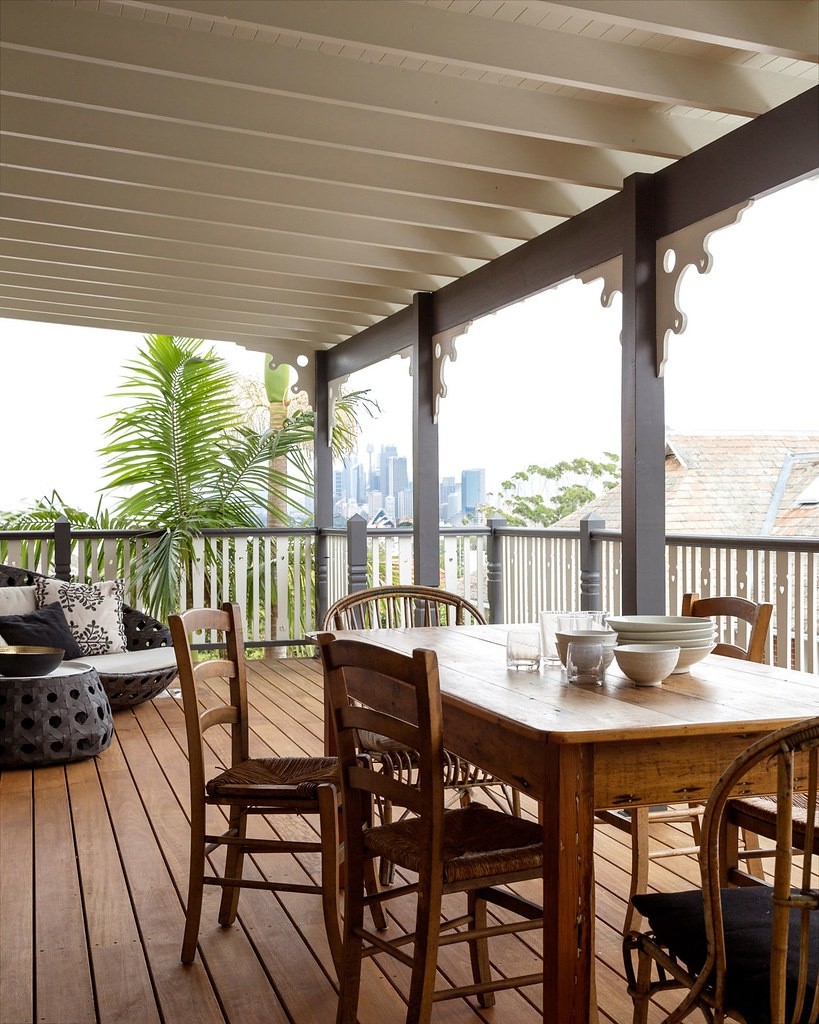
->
[0,601,87,663]
[33,576,130,658]
[0,584,37,647]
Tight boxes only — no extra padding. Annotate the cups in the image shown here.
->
[567,642,605,688]
[506,631,541,672]
[540,611,610,667]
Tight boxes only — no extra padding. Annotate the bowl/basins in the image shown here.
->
[605,616,719,675]
[613,644,681,686]
[0,646,65,677]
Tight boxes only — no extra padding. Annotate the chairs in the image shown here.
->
[624,713,819,1024]
[166,599,389,984]
[321,585,521,885]
[0,565,181,714]
[312,631,543,1024]
[593,588,774,938]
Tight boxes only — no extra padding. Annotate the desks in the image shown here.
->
[302,620,819,1024]
